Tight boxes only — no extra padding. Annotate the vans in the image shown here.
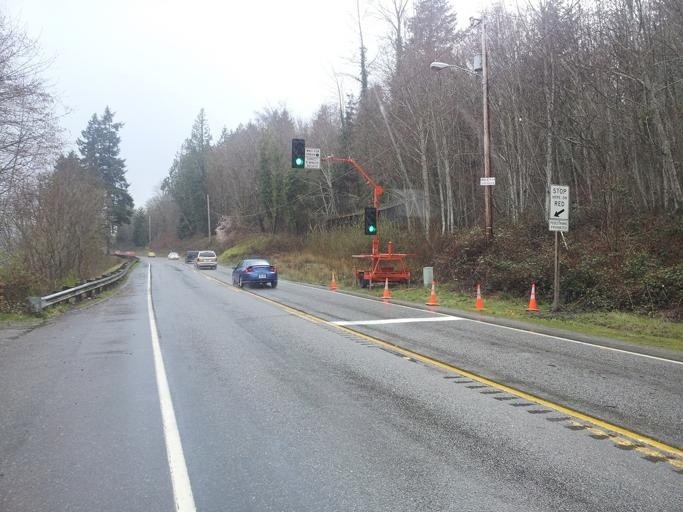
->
[194,250,218,270]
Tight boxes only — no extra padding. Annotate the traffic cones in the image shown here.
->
[380,276,392,300]
[424,279,440,307]
[524,283,539,312]
[328,272,337,289]
[472,283,485,309]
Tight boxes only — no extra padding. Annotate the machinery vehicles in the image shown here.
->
[321,154,415,289]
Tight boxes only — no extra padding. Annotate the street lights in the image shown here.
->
[429,61,493,236]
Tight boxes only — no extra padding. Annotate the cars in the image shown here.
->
[108,250,135,257]
[167,251,179,260]
[147,251,155,258]
[184,250,199,264]
[231,257,278,289]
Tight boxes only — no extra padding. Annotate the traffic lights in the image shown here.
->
[364,207,377,236]
[291,138,305,169]
[549,184,569,233]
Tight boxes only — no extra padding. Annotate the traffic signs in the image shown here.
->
[304,147,321,170]
